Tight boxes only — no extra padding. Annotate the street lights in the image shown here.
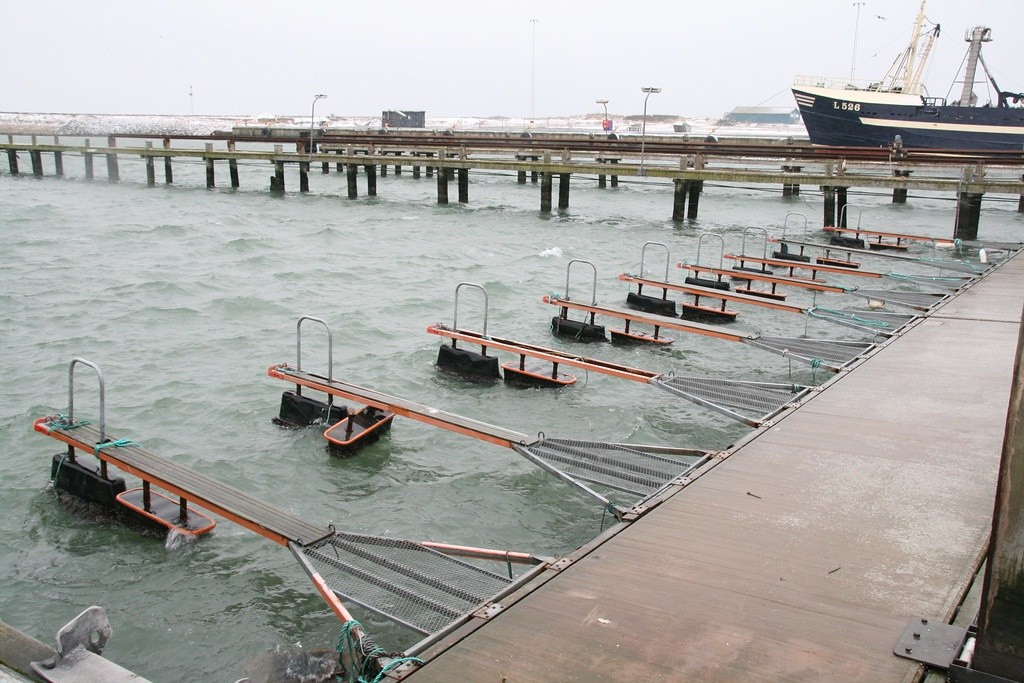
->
[529,17,541,130]
[595,99,609,137]
[309,94,328,160]
[639,86,663,174]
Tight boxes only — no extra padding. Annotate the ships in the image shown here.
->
[790,0,1024,155]
